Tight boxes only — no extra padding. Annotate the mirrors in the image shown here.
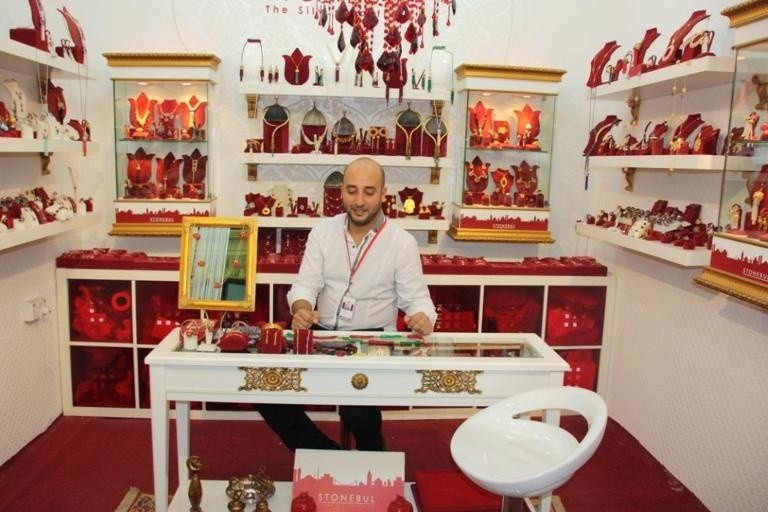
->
[178,215,258,312]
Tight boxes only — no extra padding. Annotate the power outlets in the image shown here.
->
[23,297,46,323]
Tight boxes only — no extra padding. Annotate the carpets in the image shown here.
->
[116,487,173,512]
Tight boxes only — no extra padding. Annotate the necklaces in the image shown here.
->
[498,170,510,195]
[517,162,537,191]
[127,100,202,187]
[472,112,535,147]
[2,1,93,224]
[468,163,489,182]
[590,12,713,241]
[240,41,448,218]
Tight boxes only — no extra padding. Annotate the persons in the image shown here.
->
[253,155,440,452]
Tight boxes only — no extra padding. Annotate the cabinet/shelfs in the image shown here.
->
[53,266,615,421]
[446,64,567,241]
[103,52,220,237]
[574,54,736,265]
[0,36,103,252]
[692,0,768,308]
[237,84,450,255]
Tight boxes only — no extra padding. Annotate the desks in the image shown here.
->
[142,323,571,512]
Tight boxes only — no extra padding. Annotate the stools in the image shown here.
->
[449,385,607,512]
